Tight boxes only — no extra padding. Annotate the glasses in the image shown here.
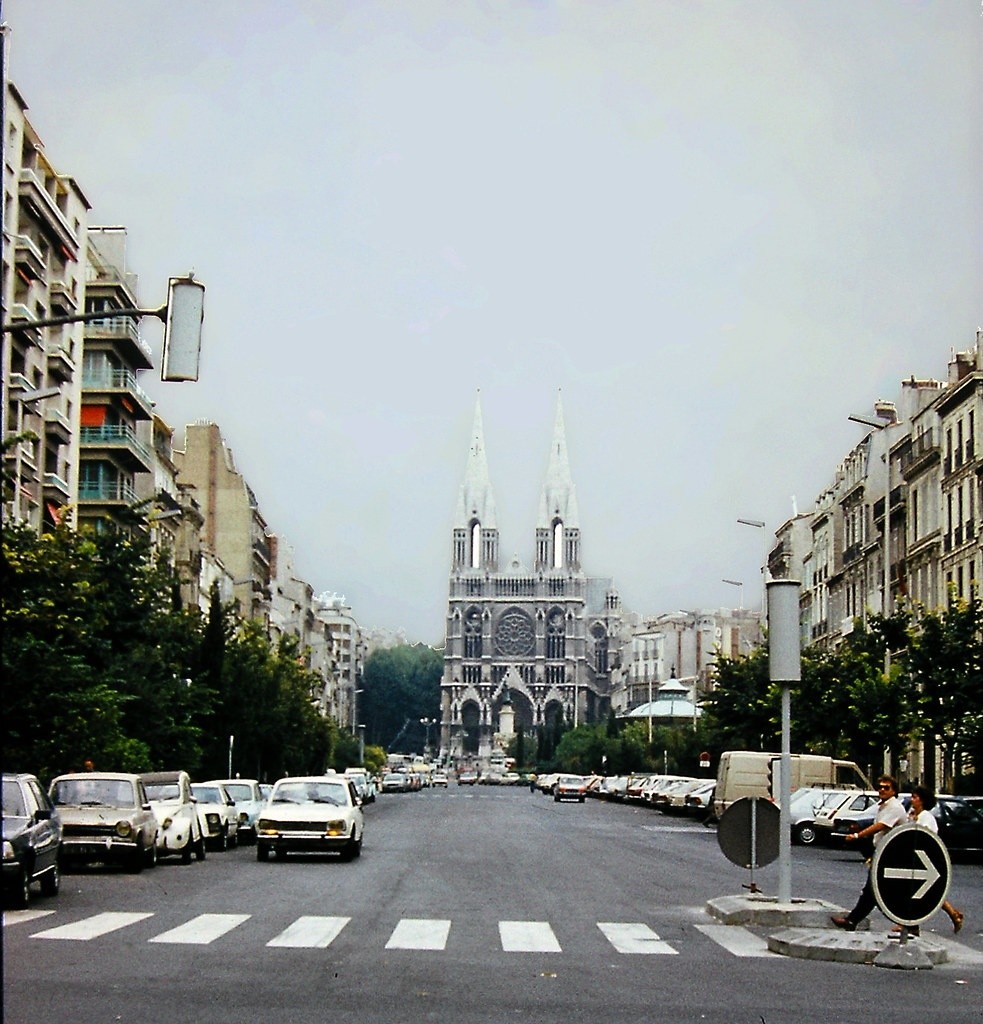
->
[878,785,891,791]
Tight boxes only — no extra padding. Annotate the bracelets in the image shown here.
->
[854,832,858,838]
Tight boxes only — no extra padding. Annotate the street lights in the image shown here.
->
[846,413,892,776]
[736,517,766,651]
[352,689,365,739]
[357,724,366,763]
[721,578,744,659]
[419,718,438,748]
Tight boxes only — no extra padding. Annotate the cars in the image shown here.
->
[45,770,159,874]
[551,775,586,803]
[189,781,239,853]
[325,753,448,806]
[203,779,266,846]
[773,786,983,865]
[253,776,365,864]
[454,756,718,819]
[116,770,206,865]
[1,772,63,910]
[458,773,474,787]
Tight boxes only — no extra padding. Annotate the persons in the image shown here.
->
[529,772,537,793]
[829,774,964,936]
[702,774,719,828]
[68,758,95,773]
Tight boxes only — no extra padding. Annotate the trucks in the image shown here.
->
[713,750,877,822]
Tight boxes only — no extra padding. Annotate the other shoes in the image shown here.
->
[953,911,963,933]
[831,917,856,932]
